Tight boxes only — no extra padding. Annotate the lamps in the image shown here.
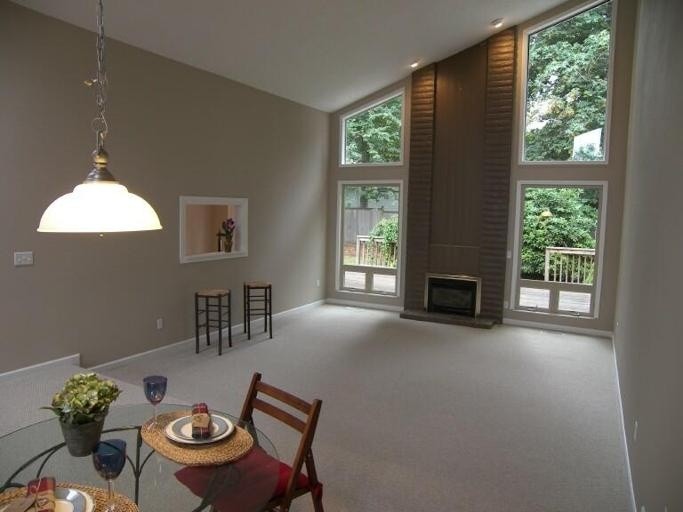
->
[36,0,164,237]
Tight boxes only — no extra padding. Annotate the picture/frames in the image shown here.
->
[177,195,250,265]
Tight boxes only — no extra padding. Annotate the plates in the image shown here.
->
[160,414,236,445]
[0,487,96,512]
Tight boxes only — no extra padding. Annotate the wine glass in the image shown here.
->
[91,438,128,512]
[143,374,167,433]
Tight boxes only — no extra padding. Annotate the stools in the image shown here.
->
[194,281,273,356]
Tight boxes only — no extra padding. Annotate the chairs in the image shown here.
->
[174,370,324,511]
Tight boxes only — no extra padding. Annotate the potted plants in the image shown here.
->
[40,370,124,456]
